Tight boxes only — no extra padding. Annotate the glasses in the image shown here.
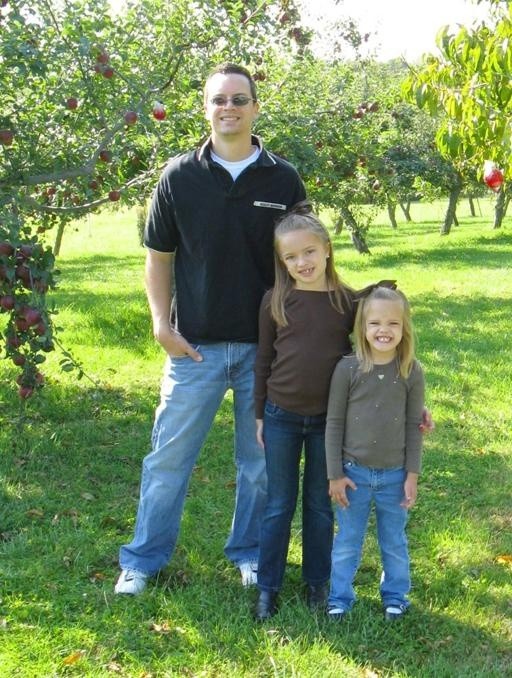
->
[209,95,258,107]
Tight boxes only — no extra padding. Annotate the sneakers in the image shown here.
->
[306,581,327,612]
[254,589,277,624]
[113,567,150,596]
[383,606,406,625]
[238,561,260,588]
[324,608,345,625]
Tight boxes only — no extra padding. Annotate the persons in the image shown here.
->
[251,198,436,623]
[114,60,312,596]
[321,279,426,624]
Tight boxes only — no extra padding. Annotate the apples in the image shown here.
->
[100,150,113,163]
[484,168,504,189]
[254,57,262,65]
[1,241,49,347]
[153,107,166,121]
[109,191,121,201]
[0,128,13,146]
[94,52,113,78]
[280,12,304,55]
[126,111,137,124]
[14,354,26,366]
[34,186,80,233]
[90,174,103,188]
[252,70,266,82]
[315,99,401,190]
[240,13,248,24]
[16,373,43,397]
[67,98,77,109]
